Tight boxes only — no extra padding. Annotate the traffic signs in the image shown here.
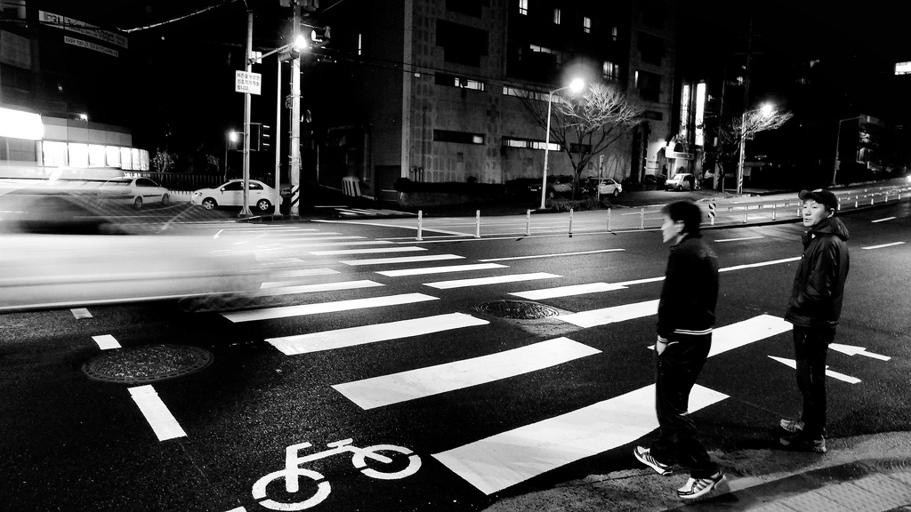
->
[226,130,240,147]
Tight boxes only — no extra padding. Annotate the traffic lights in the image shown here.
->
[300,25,330,52]
[259,122,270,152]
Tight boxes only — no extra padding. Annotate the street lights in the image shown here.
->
[238,34,308,215]
[735,103,772,198]
[831,115,864,186]
[538,77,584,209]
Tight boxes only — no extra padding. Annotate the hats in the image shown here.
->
[797,187,837,211]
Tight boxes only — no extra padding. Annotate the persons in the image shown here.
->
[661,164,711,192]
[778,188,850,453]
[633,200,728,499]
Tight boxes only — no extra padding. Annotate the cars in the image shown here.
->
[505,175,624,198]
[663,172,699,191]
[0,174,286,313]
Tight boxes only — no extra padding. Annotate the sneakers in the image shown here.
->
[777,431,826,453]
[676,471,726,500]
[633,445,673,475]
[779,417,824,438]
